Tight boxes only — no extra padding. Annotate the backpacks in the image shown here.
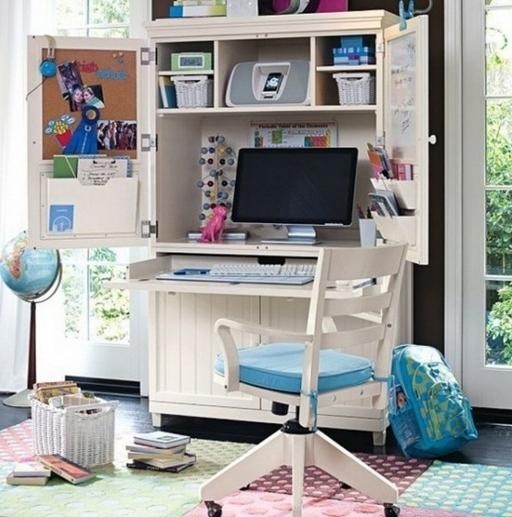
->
[386,342,478,460]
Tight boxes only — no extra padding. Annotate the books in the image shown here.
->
[365,141,417,221]
[124,429,197,475]
[7,451,95,486]
[34,378,102,416]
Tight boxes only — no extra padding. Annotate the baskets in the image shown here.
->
[28,383,120,466]
[334,73,375,105]
[173,80,213,108]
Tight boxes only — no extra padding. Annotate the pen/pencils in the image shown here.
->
[357,201,371,219]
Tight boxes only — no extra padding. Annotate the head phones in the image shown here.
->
[38,34,58,78]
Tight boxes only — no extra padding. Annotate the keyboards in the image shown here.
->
[209,262,317,278]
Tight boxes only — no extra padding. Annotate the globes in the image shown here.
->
[0,229,62,408]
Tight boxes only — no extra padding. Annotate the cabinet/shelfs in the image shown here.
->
[26,10,430,268]
[115,249,409,444]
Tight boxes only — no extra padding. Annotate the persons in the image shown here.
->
[81,88,105,109]
[72,87,84,110]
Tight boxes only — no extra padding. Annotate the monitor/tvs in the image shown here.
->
[228,147,358,245]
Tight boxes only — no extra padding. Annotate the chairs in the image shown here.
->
[197,242,412,517]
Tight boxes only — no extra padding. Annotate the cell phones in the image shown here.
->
[261,72,284,94]
